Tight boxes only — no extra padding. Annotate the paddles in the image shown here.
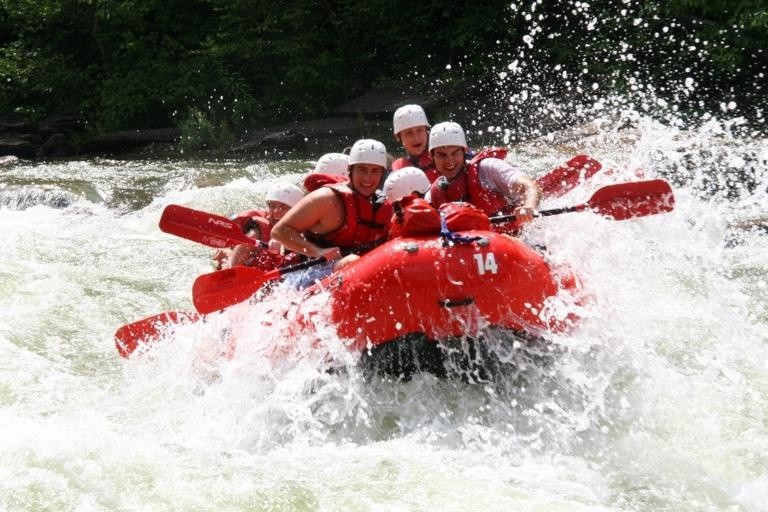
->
[159,204,295,264]
[490,155,601,214]
[115,310,201,358]
[489,179,674,224]
[192,239,385,314]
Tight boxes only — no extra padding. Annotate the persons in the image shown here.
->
[424,121,542,233]
[375,167,477,212]
[214,152,350,270]
[389,104,440,183]
[272,139,393,293]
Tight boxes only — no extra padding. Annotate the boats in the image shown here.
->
[206,231,594,385]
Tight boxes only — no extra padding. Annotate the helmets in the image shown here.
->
[266,184,305,208]
[315,105,465,204]
